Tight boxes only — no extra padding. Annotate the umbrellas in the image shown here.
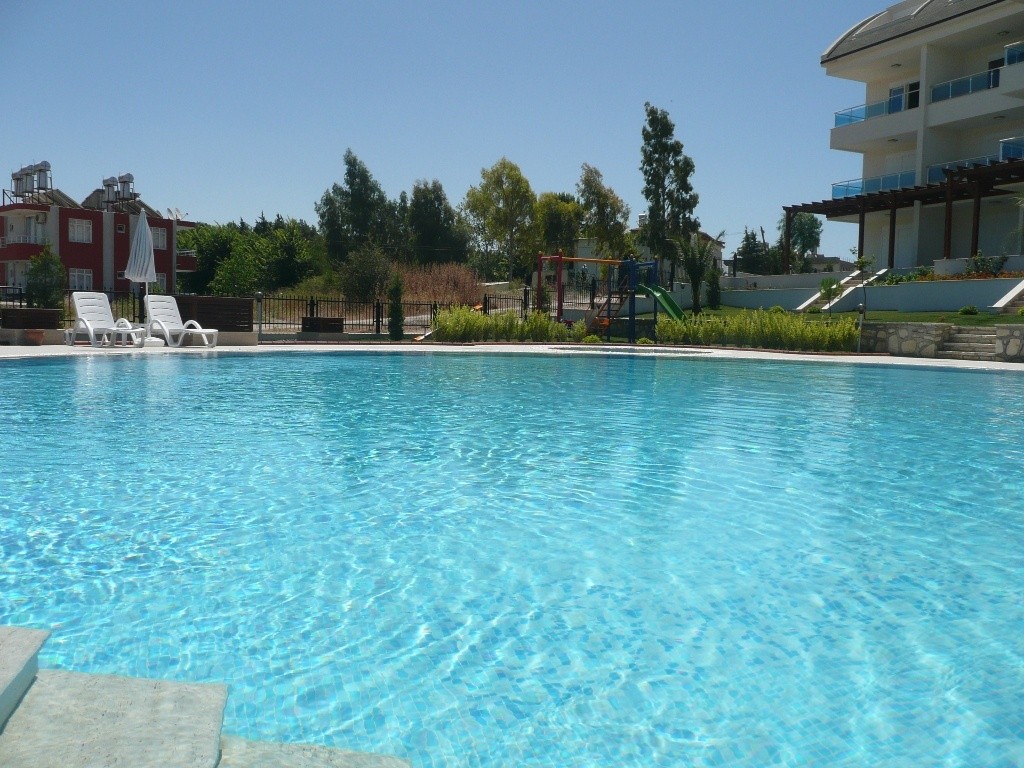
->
[120,207,166,337]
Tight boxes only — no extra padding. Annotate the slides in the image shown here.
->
[638,282,688,324]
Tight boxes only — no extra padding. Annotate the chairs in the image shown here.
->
[141,295,219,348]
[66,291,145,347]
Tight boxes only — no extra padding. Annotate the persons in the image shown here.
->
[581,263,588,281]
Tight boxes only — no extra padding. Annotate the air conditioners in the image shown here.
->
[35,213,46,223]
